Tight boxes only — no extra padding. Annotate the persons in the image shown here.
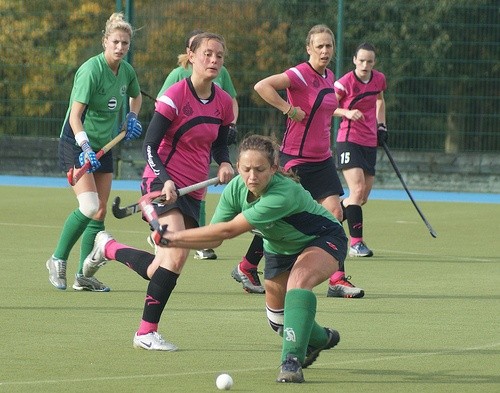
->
[147,134,347,384]
[83,33,234,352]
[231,26,365,298]
[331,43,388,254]
[155,31,239,260]
[46,12,142,292]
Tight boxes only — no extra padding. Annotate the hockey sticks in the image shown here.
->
[67,131,126,186]
[139,190,168,246]
[382,141,437,238]
[141,90,157,102]
[112,176,220,219]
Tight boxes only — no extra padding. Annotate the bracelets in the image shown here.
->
[288,107,298,118]
[74,131,89,147]
[283,105,292,115]
[377,122,386,128]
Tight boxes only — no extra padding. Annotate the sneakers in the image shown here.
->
[349,240,373,257]
[302,326,340,368]
[277,352,305,383]
[231,261,266,294]
[73,273,110,292]
[82,231,113,278]
[193,248,217,261]
[134,330,175,352]
[327,276,364,297]
[46,252,67,290]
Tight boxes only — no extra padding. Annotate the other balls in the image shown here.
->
[216,374,233,390]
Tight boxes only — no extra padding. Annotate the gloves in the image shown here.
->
[120,112,142,142]
[79,141,102,172]
[377,123,388,146]
[226,121,238,146]
